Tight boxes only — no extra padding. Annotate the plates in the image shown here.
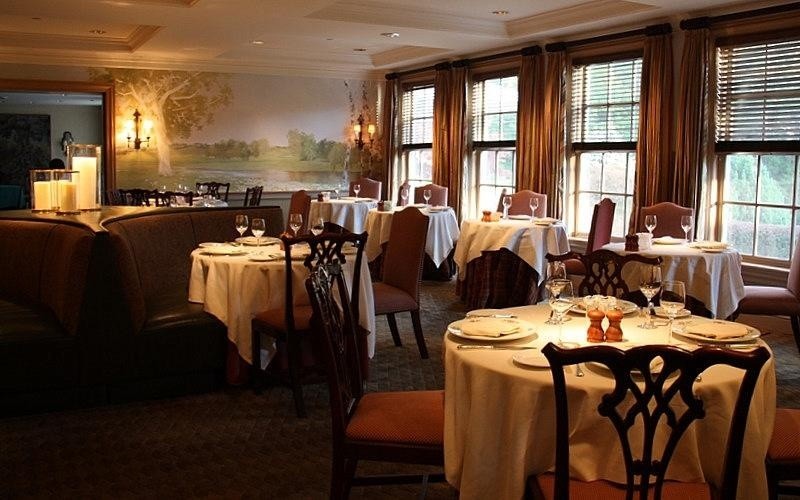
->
[670,319,762,342]
[589,342,664,374]
[466,308,519,319]
[406,202,451,212]
[653,236,684,244]
[513,350,551,368]
[643,305,692,318]
[534,217,559,226]
[568,296,638,316]
[198,211,357,262]
[693,240,729,253]
[447,320,538,342]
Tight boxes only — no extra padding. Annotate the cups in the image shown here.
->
[636,232,652,251]
[490,212,500,221]
[320,192,331,201]
[65,143,103,211]
[28,167,82,215]
[149,198,156,207]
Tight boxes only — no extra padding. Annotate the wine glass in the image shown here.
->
[644,215,657,245]
[639,265,662,329]
[680,215,694,248]
[502,195,540,223]
[423,189,433,211]
[333,183,373,202]
[400,188,409,208]
[157,182,216,207]
[542,258,577,350]
[658,279,686,345]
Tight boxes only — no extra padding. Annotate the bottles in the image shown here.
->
[625,234,639,252]
[585,306,624,342]
[317,192,324,202]
[377,200,392,211]
[481,211,492,222]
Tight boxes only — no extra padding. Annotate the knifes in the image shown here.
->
[457,344,537,351]
[697,344,760,350]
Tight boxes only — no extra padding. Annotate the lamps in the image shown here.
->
[122,108,154,152]
[354,114,376,151]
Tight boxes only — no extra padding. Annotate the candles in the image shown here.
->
[29,147,100,211]
